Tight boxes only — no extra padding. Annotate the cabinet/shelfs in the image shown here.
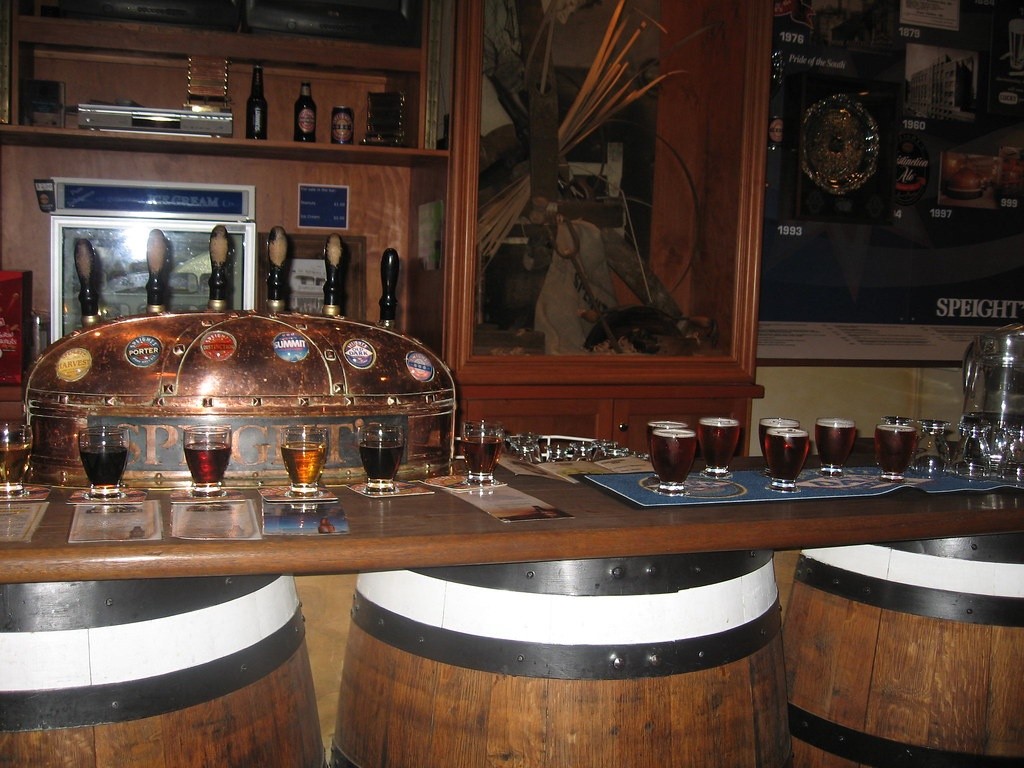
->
[408,0,772,457]
[0,0,466,482]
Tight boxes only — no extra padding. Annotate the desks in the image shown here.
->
[0,450,1024,768]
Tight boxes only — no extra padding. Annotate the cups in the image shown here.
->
[880,416,926,469]
[951,421,992,480]
[765,428,810,493]
[759,418,800,475]
[0,422,33,499]
[461,418,503,486]
[1009,19,1024,69]
[996,425,1024,482]
[874,424,917,482]
[651,429,696,495]
[78,427,130,501]
[909,420,951,479]
[358,423,405,495]
[280,428,329,499]
[183,426,232,497]
[698,418,739,479]
[647,421,689,481]
[814,417,856,476]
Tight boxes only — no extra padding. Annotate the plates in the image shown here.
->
[947,187,983,194]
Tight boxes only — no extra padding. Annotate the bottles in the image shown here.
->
[245,64,268,140]
[293,79,317,142]
